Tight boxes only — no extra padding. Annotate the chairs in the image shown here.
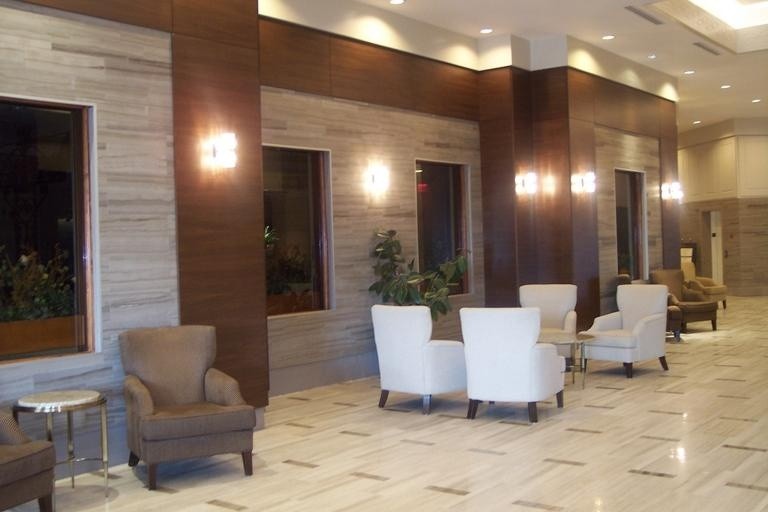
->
[369,304,468,416]
[459,307,566,423]
[581,284,668,378]
[650,268,718,334]
[519,283,577,372]
[681,260,727,309]
[118,325,256,490]
[0,406,56,512]
[616,273,682,343]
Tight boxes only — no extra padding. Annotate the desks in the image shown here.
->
[13,390,109,497]
[536,333,594,390]
